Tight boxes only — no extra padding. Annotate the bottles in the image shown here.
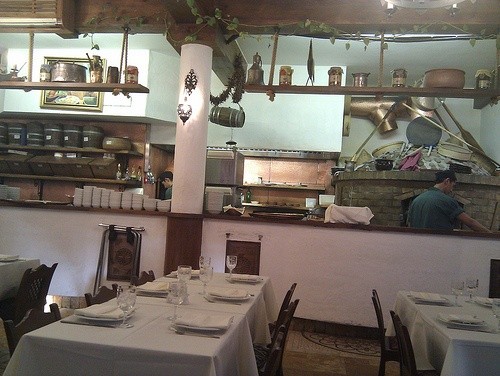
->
[166,279,189,306]
[345,161,354,172]
[40,64,138,84]
[279,65,492,90]
[246,188,251,203]
[203,186,232,213]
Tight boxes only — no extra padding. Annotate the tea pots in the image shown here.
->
[248,52,264,85]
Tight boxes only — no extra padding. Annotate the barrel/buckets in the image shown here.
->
[0,121,104,149]
[209,106,246,128]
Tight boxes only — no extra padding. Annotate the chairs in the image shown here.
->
[252,283,299,376]
[85,222,155,306]
[370,290,439,376]
[0,262,61,359]
[224,233,264,276]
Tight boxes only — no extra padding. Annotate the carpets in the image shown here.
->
[385,290,500,376]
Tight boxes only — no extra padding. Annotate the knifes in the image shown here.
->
[60,320,115,328]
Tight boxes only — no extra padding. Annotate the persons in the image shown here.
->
[405,170,494,233]
[157,171,173,200]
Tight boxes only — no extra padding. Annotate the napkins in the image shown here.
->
[209,285,249,298]
[223,272,263,282]
[137,280,169,290]
[0,254,19,260]
[440,313,481,323]
[175,310,235,330]
[76,302,134,319]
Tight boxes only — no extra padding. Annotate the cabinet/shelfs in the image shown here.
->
[241,184,325,211]
[0,144,144,187]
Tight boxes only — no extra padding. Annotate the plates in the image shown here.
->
[0,185,21,200]
[170,272,200,276]
[306,194,336,209]
[0,258,20,261]
[407,292,500,334]
[135,288,169,293]
[73,310,135,321]
[176,276,264,331]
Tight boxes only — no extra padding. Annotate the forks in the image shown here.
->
[170,327,221,339]
[204,296,242,305]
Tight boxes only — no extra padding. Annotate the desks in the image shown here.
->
[2,269,277,376]
[0,258,41,302]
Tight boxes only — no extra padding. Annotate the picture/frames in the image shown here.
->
[41,57,107,112]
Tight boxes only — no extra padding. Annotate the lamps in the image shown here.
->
[178,70,198,125]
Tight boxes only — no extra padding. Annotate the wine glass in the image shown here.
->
[176,255,237,296]
[115,284,136,329]
[449,275,500,333]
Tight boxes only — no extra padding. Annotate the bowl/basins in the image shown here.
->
[423,68,465,88]
[65,185,171,212]
[375,158,393,170]
[102,137,132,150]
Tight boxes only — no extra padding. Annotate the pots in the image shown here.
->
[50,63,87,83]
